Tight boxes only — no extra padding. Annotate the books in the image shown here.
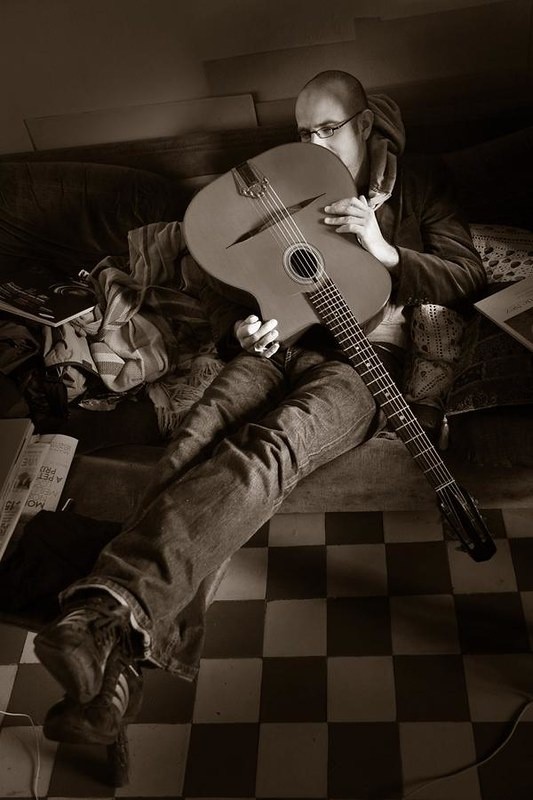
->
[0,419,35,515]
[0,281,96,327]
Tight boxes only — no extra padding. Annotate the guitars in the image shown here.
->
[182,141,500,565]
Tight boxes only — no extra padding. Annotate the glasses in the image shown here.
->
[298,110,363,143]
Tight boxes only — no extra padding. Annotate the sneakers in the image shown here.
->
[42,655,144,747]
[33,590,132,702]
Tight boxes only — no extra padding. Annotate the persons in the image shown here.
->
[33,70,488,788]
